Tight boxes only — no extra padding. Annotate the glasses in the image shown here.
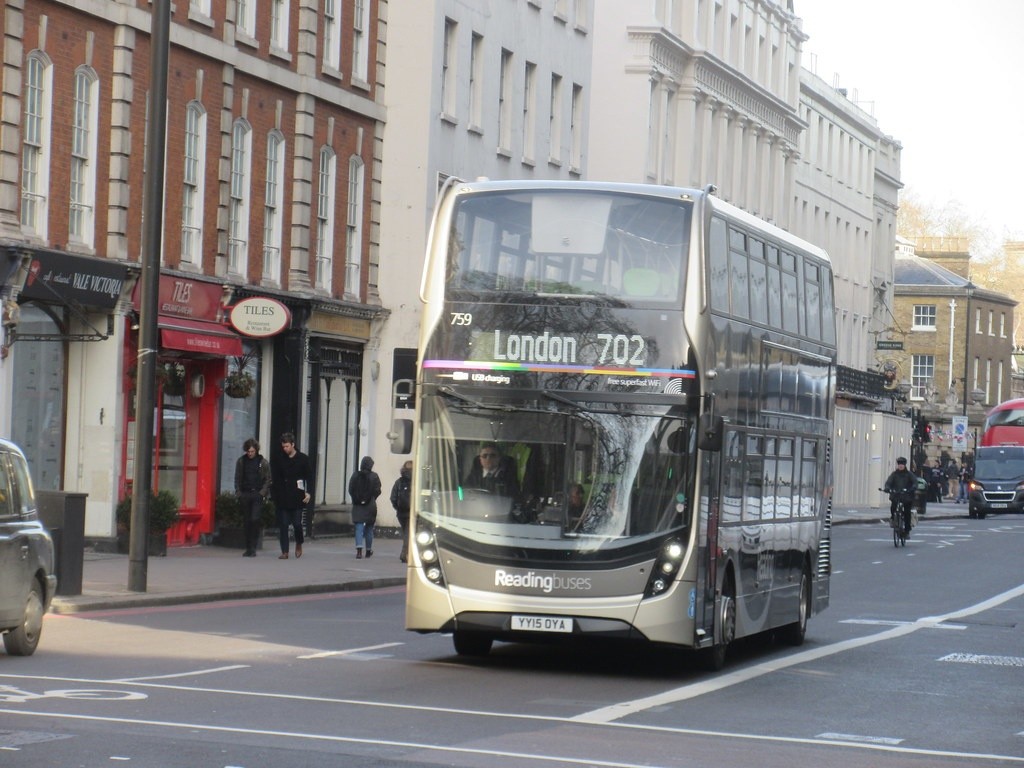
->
[480,454,496,459]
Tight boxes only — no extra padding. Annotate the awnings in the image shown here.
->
[158,314,241,356]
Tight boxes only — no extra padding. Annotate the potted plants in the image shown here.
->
[116,489,180,557]
[214,491,276,549]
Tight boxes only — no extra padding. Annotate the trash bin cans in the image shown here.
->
[913,479,928,515]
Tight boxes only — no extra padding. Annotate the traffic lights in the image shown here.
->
[924,425,931,442]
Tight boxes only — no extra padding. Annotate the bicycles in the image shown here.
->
[878,488,906,547]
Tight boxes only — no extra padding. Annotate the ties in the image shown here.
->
[485,473,491,481]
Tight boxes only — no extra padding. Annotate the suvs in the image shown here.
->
[969,446,1024,519]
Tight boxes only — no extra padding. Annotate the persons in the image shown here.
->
[270,433,313,558]
[349,456,382,558]
[885,458,917,539]
[568,484,586,518]
[233,440,271,555]
[465,443,517,498]
[391,461,412,561]
[915,459,972,505]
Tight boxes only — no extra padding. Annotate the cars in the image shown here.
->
[1,438,57,657]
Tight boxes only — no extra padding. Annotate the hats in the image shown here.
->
[897,457,906,463]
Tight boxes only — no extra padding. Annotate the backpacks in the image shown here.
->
[352,471,372,497]
[396,478,410,510]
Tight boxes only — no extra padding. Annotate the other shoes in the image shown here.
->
[243,551,256,557]
[295,544,302,557]
[400,552,406,562]
[366,551,373,558]
[279,553,287,558]
[356,554,362,558]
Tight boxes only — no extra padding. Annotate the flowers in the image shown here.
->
[216,345,260,399]
[153,356,187,396]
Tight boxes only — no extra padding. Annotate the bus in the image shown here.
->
[403,177,836,674]
[980,398,1024,446]
